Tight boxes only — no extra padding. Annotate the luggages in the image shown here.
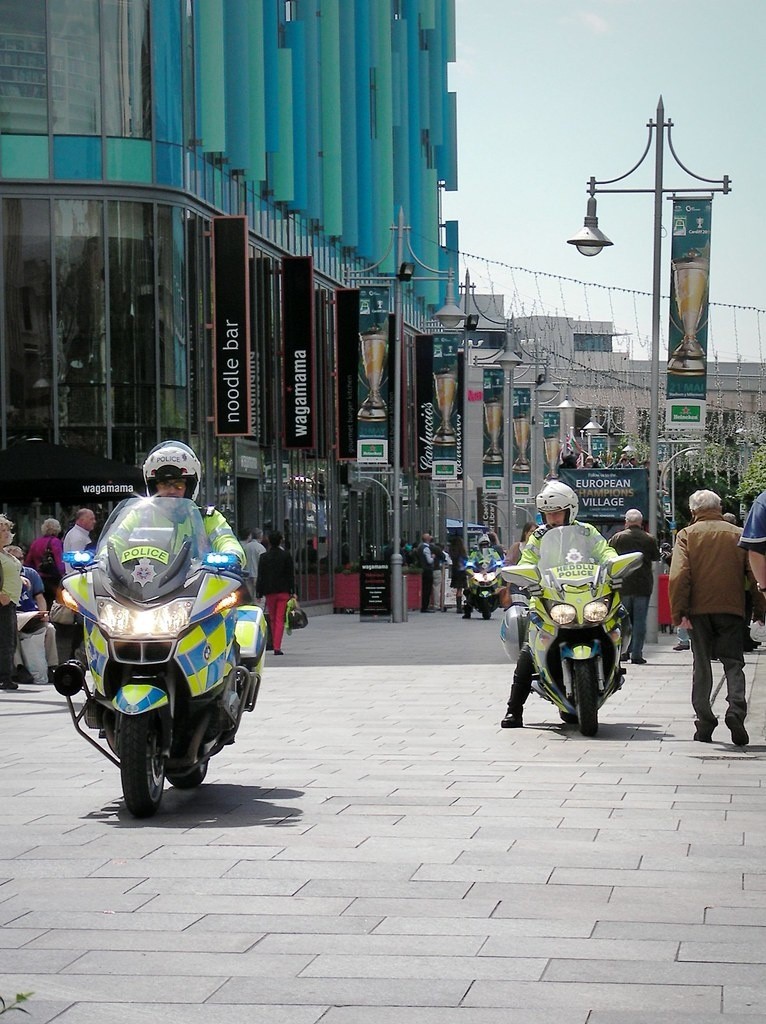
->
[658,569,675,634]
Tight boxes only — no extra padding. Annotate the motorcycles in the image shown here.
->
[497,525,644,735]
[52,497,268,820]
[459,547,504,619]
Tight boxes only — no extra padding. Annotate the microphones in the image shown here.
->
[551,523,570,527]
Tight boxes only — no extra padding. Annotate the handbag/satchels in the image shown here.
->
[285,598,309,636]
[38,537,58,575]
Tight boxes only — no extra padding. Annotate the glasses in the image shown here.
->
[156,480,188,490]
[16,556,25,561]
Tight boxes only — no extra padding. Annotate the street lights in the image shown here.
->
[474,312,560,550]
[598,404,637,468]
[509,334,581,496]
[420,268,522,553]
[539,378,581,481]
[344,205,468,623]
[565,393,604,450]
[568,96,731,643]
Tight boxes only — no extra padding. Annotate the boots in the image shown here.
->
[456,595,463,613]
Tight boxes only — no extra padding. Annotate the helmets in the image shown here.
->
[536,482,579,525]
[478,537,491,550]
[143,441,202,501]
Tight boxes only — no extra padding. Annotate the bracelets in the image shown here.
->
[757,582,766,593]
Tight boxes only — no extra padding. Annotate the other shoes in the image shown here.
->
[725,713,749,747]
[674,644,690,651]
[421,608,435,613]
[631,658,647,664]
[48,665,57,682]
[17,664,34,683]
[274,650,283,655]
[621,652,630,661]
[694,731,712,743]
[0,679,19,689]
[201,708,221,745]
[462,614,471,619]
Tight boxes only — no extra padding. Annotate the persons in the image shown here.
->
[0,507,96,691]
[668,489,766,746]
[607,509,671,664]
[237,527,298,656]
[400,532,507,619]
[559,448,638,468]
[91,439,247,666]
[501,478,632,728]
[500,522,539,609]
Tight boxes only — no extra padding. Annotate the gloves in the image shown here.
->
[203,551,242,575]
[72,550,96,567]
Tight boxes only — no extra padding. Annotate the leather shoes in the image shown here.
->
[501,712,523,727]
